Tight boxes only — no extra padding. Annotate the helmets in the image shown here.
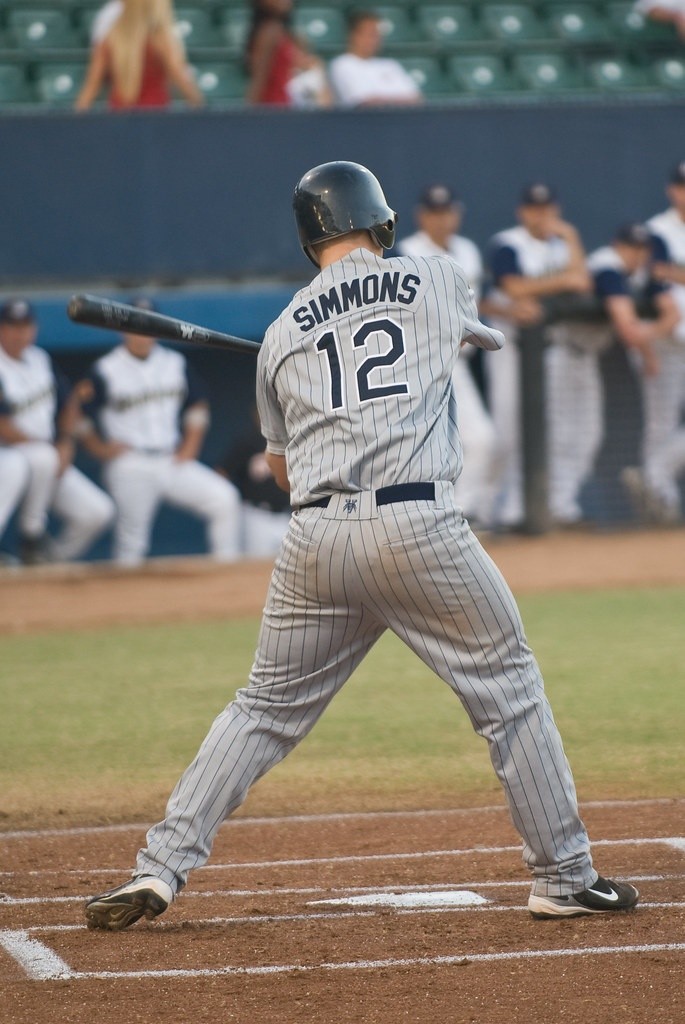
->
[292,161,398,269]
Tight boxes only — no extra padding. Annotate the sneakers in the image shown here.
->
[84,875,173,931]
[528,874,639,917]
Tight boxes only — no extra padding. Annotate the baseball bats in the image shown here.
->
[64,292,262,352]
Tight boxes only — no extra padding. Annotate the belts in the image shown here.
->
[300,482,435,509]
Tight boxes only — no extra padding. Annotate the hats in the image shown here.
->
[523,184,556,203]
[619,224,650,245]
[0,299,31,323]
[418,184,454,209]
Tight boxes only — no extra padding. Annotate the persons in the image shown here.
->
[87,158,641,933]
[0,0,685,572]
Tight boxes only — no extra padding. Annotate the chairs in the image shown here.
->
[0,0,685,110]
